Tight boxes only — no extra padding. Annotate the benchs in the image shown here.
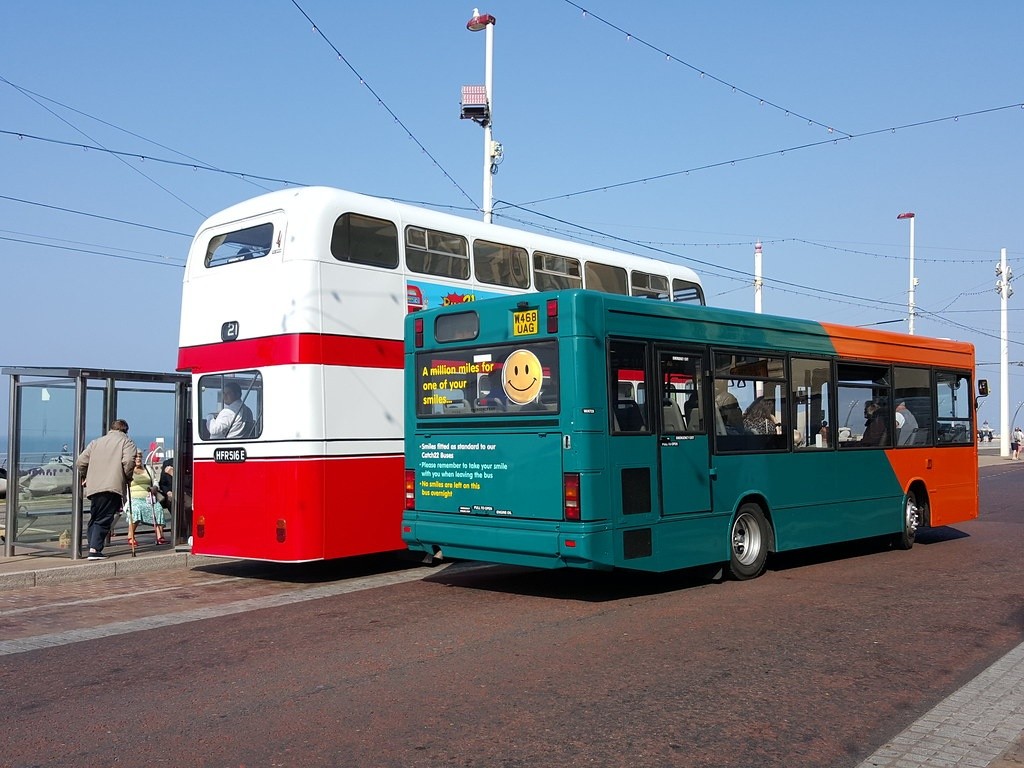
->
[16,505,125,542]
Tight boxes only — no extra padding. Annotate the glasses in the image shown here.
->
[136,456,142,458]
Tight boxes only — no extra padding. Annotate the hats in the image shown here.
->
[714,392,739,406]
[1015,427,1019,430]
[136,448,143,454]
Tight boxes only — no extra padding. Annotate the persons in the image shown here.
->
[126,448,171,545]
[61,444,69,456]
[483,368,506,407]
[159,458,174,514]
[684,378,919,445]
[980,431,983,442]
[206,383,254,438]
[75,419,137,561]
[1016,429,1024,460]
[988,432,993,442]
[1011,427,1020,460]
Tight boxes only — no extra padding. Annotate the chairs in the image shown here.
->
[904,427,932,445]
[434,239,467,278]
[745,428,759,435]
[481,249,561,291]
[643,398,687,431]
[614,400,646,432]
[879,427,902,446]
[688,402,727,436]
[392,228,434,273]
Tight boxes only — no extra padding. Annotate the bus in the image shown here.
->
[401,287,990,583]
[170,185,707,566]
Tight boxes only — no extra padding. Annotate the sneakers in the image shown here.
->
[88,552,109,560]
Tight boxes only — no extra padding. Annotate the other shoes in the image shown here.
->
[128,537,139,547]
[157,536,171,545]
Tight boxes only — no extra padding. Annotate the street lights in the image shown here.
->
[896,212,920,335]
[467,13,505,224]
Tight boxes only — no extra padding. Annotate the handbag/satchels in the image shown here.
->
[146,492,156,504]
[160,497,170,509]
